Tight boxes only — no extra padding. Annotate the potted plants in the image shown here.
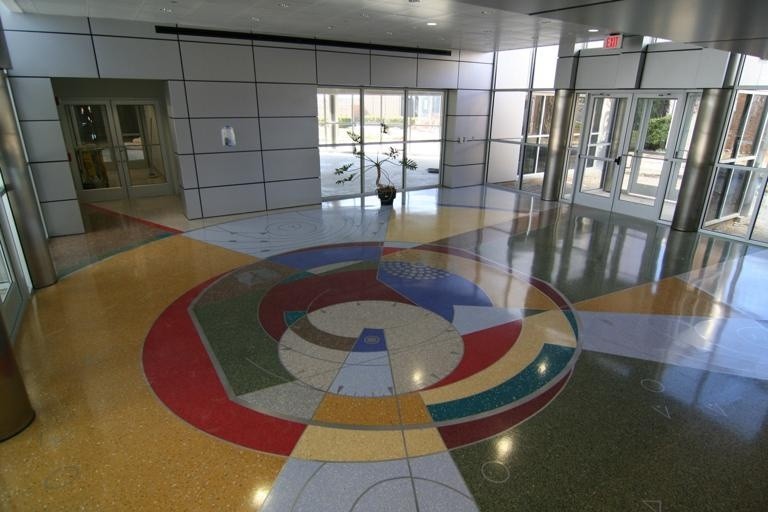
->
[335,146,417,206]
